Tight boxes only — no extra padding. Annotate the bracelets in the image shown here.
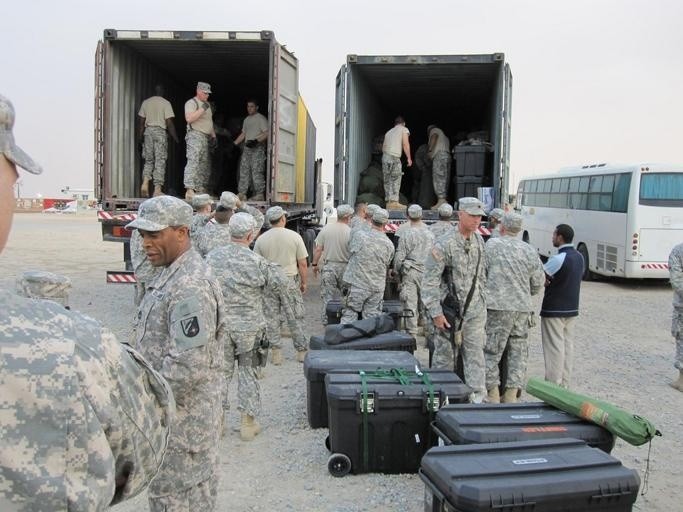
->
[312,264,317,266]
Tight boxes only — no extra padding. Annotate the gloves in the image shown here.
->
[208,137,259,149]
[201,102,209,112]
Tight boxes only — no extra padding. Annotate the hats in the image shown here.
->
[229,212,257,234]
[367,204,381,218]
[266,206,287,221]
[427,125,436,136]
[337,204,354,218]
[489,208,503,220]
[497,213,524,233]
[220,192,240,210]
[197,81,213,94]
[125,194,193,232]
[438,203,452,216]
[408,204,422,217]
[372,208,390,224]
[0,93,45,175]
[459,197,488,217]
[194,193,211,206]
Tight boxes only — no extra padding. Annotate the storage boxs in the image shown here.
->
[451,142,486,207]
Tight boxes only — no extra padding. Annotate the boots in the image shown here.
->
[220,408,263,442]
[482,386,519,404]
[270,346,306,366]
[668,368,683,393]
[140,177,165,198]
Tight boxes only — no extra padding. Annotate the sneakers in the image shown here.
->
[385,201,408,210]
[405,332,433,346]
[431,199,448,210]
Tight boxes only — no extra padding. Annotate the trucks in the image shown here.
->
[329,41,515,239]
[88,21,332,292]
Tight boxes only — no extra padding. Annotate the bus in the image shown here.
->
[516,157,683,284]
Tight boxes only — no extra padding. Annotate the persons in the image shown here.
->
[481,203,545,405]
[0,91,175,512]
[341,202,455,345]
[130,229,164,307]
[421,197,490,404]
[123,190,233,511]
[664,240,682,394]
[538,221,584,389]
[380,115,452,211]
[188,192,290,443]
[136,79,268,202]
[312,204,354,325]
[253,205,311,364]
